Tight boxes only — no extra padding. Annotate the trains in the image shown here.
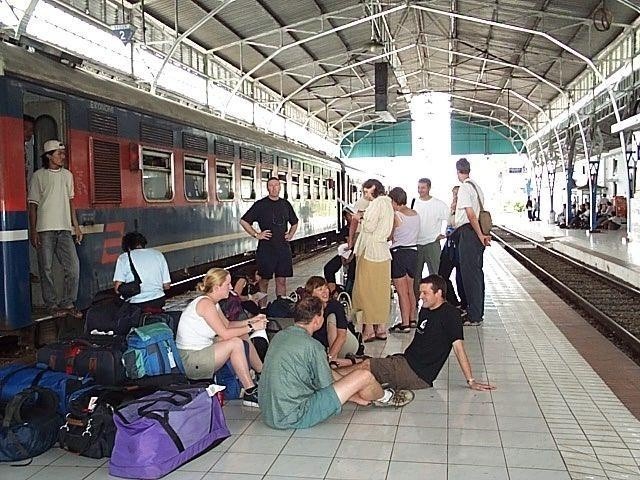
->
[0,42,395,330]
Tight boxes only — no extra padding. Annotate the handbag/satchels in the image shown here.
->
[117,280,141,299]
[220,287,306,340]
[480,210,493,236]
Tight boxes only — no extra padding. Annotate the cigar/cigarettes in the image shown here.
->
[76,240,81,245]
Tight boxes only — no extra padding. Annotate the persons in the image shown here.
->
[257,298,414,430]
[241,176,298,297]
[112,231,172,314]
[24,112,37,188]
[526,196,540,221]
[172,268,262,407]
[230,264,267,314]
[557,188,622,230]
[333,275,496,391]
[456,157,492,322]
[23,138,83,320]
[306,276,370,369]
[321,178,467,344]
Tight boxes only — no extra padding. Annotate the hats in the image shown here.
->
[40,140,66,157]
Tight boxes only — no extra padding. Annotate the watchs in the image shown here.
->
[254,232,259,238]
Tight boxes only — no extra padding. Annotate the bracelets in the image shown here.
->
[247,323,254,332]
[466,378,475,383]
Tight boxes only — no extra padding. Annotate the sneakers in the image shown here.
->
[49,306,83,319]
[459,304,484,326]
[243,388,261,407]
[370,382,415,407]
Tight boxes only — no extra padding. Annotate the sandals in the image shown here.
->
[357,320,419,342]
[345,352,373,363]
